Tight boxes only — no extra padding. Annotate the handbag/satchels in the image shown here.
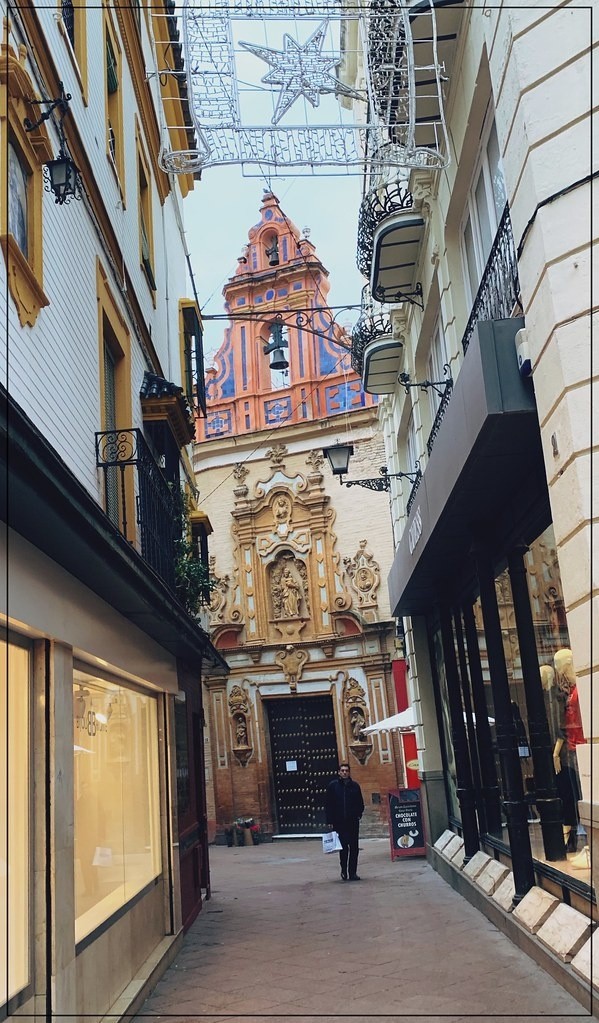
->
[322,827,343,854]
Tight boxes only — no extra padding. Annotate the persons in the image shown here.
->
[350,711,365,740]
[553,649,587,824]
[540,665,580,852]
[276,498,287,517]
[326,764,364,879]
[236,718,246,746]
[276,569,300,617]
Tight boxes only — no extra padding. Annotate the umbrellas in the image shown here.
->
[360,707,494,735]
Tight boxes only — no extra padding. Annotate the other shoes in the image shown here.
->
[349,874,361,880]
[341,870,348,879]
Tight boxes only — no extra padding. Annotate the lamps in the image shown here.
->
[21,81,85,206]
[322,440,391,494]
